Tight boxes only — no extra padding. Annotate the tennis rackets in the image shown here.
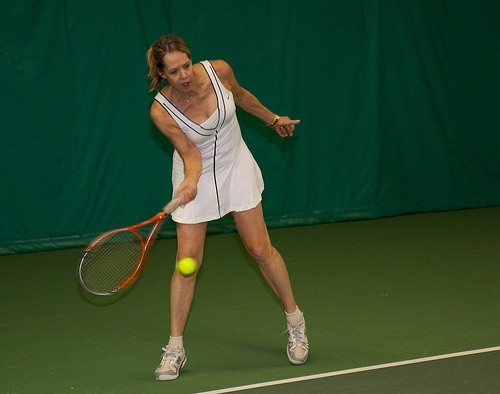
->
[78,193,185,296]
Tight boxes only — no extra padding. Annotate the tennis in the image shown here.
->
[178,257,197,275]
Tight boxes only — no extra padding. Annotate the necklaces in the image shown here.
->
[180,92,194,103]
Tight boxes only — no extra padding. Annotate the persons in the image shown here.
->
[145,36,316,382]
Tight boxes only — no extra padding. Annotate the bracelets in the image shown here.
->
[266,112,282,130]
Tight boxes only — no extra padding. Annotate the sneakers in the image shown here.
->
[286,316,310,365]
[155,345,187,381]
